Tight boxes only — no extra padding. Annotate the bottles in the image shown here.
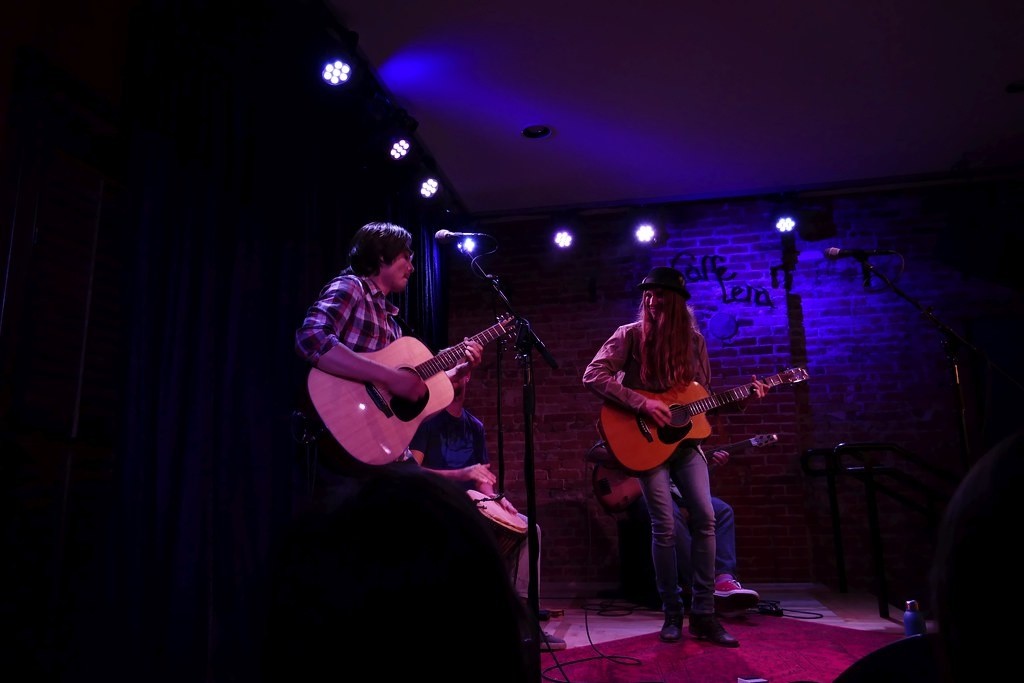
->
[904,600,926,636]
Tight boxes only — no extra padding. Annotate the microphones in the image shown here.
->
[434,229,486,244]
[826,244,873,270]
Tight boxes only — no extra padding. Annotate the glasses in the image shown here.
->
[405,244,414,262]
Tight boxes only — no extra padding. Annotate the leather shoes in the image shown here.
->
[688,610,739,647]
[659,614,684,642]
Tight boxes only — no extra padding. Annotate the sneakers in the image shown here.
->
[713,579,760,619]
[540,631,566,650]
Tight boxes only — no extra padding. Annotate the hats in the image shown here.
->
[637,267,691,300]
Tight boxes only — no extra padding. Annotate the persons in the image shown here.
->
[296,221,568,653]
[582,267,761,650]
[407,351,554,621]
[585,417,761,605]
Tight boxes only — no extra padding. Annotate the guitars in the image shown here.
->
[597,367,810,477]
[591,432,780,513]
[307,311,519,468]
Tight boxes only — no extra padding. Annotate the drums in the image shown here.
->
[464,489,527,557]
